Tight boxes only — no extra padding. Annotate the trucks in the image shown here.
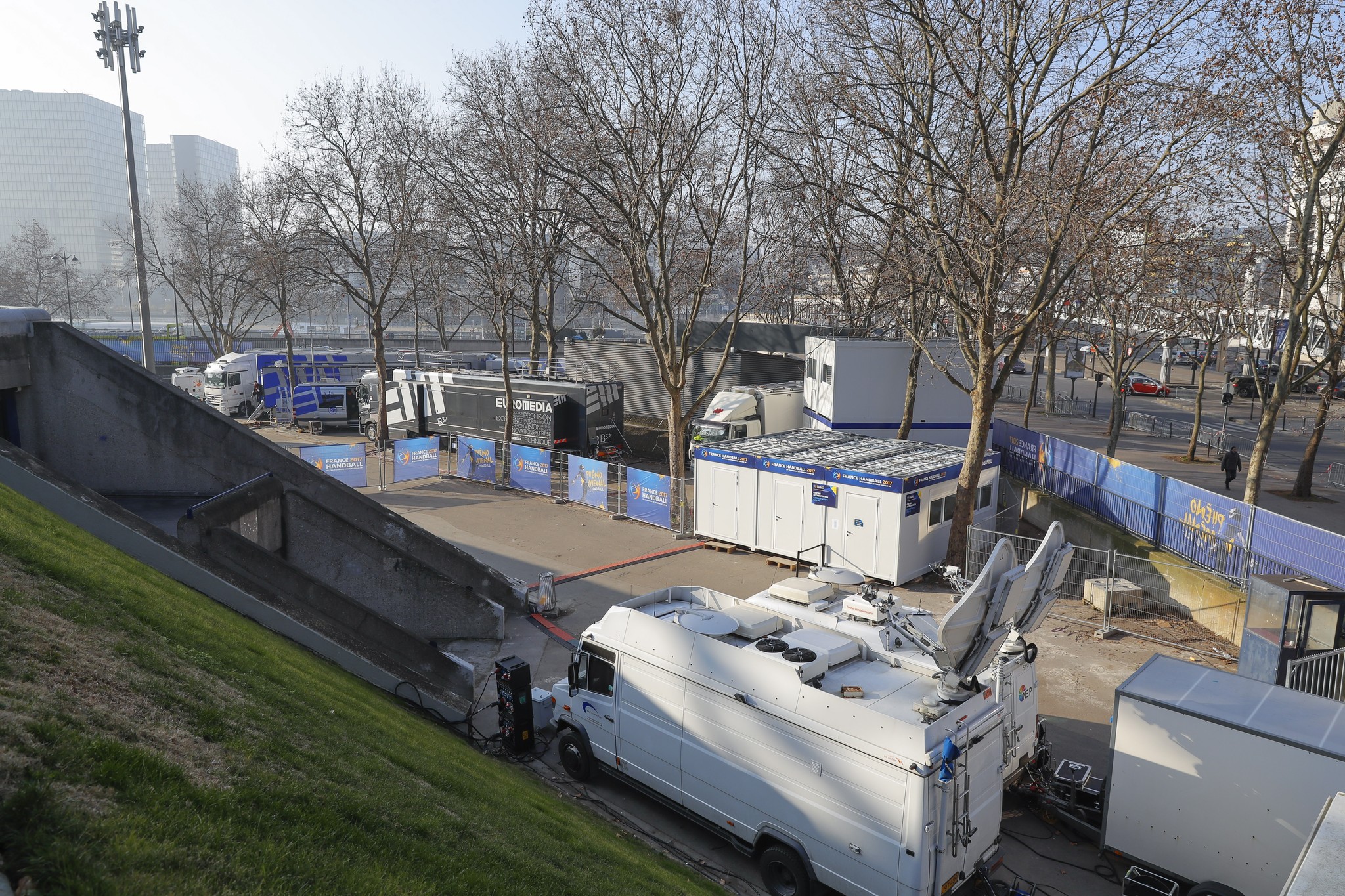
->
[687,381,804,474]
[546,520,1345,896]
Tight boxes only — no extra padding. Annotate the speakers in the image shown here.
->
[494,654,535,755]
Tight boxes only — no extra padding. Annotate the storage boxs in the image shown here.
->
[308,420,322,433]
[1053,759,1092,790]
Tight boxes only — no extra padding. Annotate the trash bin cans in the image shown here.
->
[537,572,556,610]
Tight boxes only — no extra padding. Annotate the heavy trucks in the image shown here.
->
[201,345,530,421]
[354,350,626,470]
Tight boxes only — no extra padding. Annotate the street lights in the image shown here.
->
[341,276,351,339]
[160,256,184,340]
[50,251,79,326]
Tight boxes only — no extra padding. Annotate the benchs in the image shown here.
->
[766,556,800,571]
[703,541,736,554]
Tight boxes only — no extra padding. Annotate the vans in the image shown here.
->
[291,378,361,427]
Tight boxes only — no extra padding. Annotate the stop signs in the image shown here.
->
[1091,345,1099,353]
[944,318,948,323]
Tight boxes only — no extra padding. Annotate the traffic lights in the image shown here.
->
[1191,338,1200,350]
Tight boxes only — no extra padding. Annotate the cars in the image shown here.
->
[1296,364,1322,377]
[1079,343,1110,355]
[1121,376,1170,398]
[1292,375,1328,394]
[1316,380,1345,401]
[1159,350,1217,365]
[997,355,1027,374]
[1106,370,1161,388]
[871,329,883,336]
[1241,359,1280,375]
[1229,375,1275,398]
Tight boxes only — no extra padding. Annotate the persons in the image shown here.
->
[177,386,189,394]
[346,388,357,408]
[253,380,263,406]
[1221,446,1242,490]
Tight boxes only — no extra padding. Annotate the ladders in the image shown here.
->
[602,445,630,479]
[280,390,289,421]
